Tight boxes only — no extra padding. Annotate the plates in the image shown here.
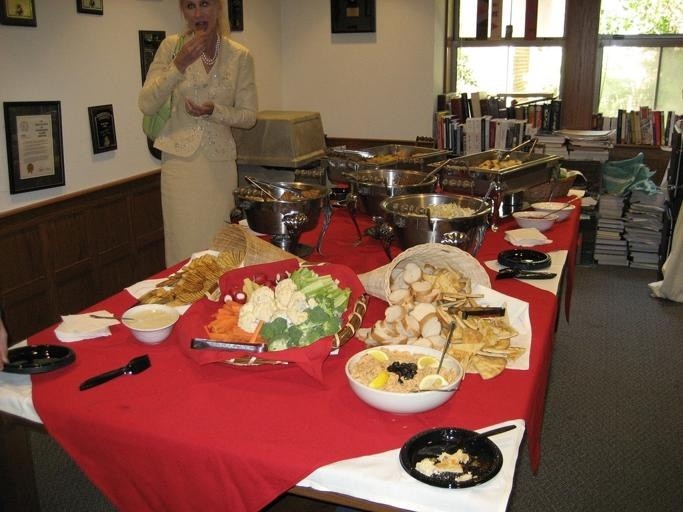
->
[1,345,76,374]
[401,427,504,487]
[499,249,552,271]
[179,262,367,365]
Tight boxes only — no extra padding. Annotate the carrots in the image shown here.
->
[205,300,263,343]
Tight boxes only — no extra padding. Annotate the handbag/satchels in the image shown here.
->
[142,36,184,142]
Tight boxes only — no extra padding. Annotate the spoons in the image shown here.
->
[494,269,558,280]
[80,354,151,394]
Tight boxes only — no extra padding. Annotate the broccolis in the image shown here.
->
[238,268,351,352]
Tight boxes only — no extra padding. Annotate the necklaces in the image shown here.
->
[192,31,220,65]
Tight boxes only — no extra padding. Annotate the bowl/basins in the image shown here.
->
[530,201,575,221]
[232,181,332,234]
[513,211,558,233]
[344,169,439,219]
[379,193,491,248]
[433,150,559,220]
[328,145,451,193]
[121,304,177,343]
[345,346,464,413]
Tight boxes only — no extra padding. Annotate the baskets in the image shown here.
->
[208,223,307,269]
[360,242,491,307]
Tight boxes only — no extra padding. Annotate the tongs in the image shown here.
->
[498,136,539,168]
[190,338,267,354]
[244,174,301,203]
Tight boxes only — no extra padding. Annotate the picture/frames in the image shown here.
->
[87,104,118,155]
[0,0,38,29]
[138,28,167,87]
[330,0,376,35]
[2,100,66,196]
[76,0,103,16]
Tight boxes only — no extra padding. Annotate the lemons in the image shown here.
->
[367,349,389,363]
[420,374,448,390]
[417,356,440,370]
[369,371,390,389]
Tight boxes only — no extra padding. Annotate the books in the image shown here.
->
[594,194,664,270]
[556,107,675,160]
[435,94,567,162]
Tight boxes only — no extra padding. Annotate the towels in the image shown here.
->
[504,227,552,247]
[56,310,120,342]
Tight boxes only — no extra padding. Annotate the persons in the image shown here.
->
[138,0,258,269]
